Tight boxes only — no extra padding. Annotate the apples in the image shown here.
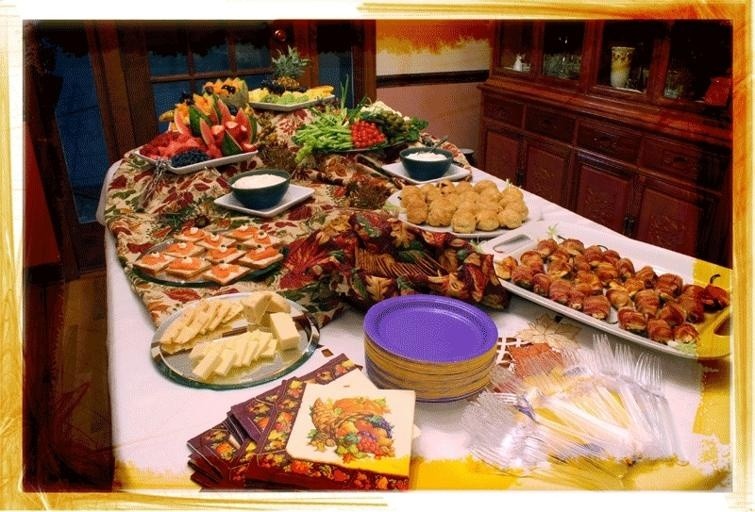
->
[355,419,373,432]
[372,427,388,438]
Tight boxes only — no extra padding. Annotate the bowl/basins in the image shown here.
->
[226,168,289,207]
[398,146,453,179]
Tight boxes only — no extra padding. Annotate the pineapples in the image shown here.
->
[271,44,311,96]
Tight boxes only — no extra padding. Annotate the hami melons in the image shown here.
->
[201,76,249,109]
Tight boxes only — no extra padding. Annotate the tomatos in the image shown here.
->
[351,119,386,147]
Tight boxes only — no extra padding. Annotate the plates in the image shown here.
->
[131,148,257,175]
[382,159,542,238]
[212,183,314,218]
[480,219,732,357]
[363,294,498,404]
[247,94,337,110]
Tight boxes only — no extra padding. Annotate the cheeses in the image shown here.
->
[160,291,299,381]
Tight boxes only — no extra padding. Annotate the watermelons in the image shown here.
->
[174,92,258,157]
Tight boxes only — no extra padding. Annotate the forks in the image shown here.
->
[459,335,689,491]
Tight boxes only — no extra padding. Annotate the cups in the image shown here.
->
[611,46,636,89]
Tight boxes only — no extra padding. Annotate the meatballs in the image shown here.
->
[397,179,529,232]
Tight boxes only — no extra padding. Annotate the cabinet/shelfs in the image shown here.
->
[477,20,732,268]
